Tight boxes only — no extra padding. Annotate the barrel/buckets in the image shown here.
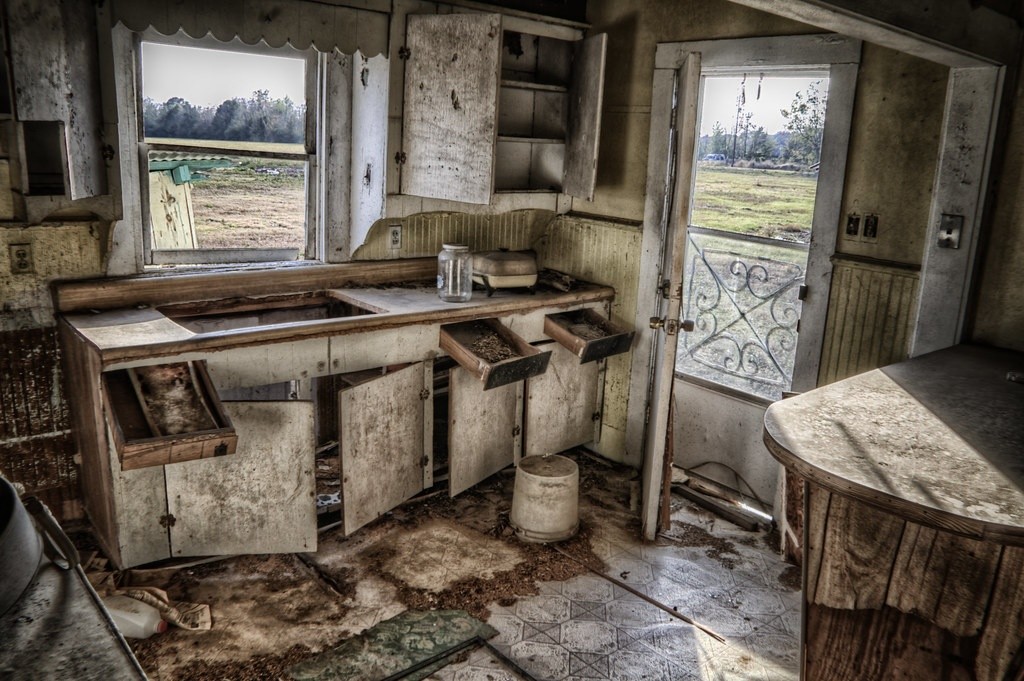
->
[509,452,582,545]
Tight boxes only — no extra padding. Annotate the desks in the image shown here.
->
[763,340,1024,681]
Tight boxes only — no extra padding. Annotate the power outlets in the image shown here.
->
[9,242,34,273]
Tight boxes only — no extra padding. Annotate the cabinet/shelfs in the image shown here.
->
[433,300,635,497]
[100,321,433,570]
[398,14,605,203]
[0,0,109,200]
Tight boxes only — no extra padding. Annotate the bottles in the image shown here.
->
[101,594,167,639]
[435,243,473,302]
